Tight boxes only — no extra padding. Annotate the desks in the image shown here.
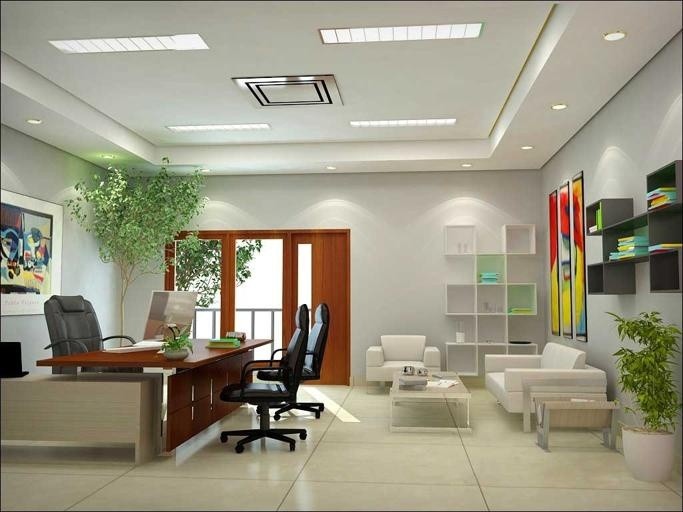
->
[0,338,273,466]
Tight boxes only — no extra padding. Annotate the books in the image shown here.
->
[208,338,237,342]
[207,341,240,348]
[511,308,534,315]
[430,378,460,390]
[588,202,602,234]
[647,243,683,251]
[607,234,648,263]
[645,184,678,211]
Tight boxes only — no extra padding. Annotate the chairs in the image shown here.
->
[42,294,142,376]
[365,334,440,393]
[218,301,330,451]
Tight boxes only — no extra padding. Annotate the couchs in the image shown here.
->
[483,342,605,432]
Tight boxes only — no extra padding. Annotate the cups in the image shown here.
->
[456,332,463,343]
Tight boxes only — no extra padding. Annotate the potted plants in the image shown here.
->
[605,308,682,482]
[161,331,194,361]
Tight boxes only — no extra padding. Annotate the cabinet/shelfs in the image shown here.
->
[585,161,681,298]
[443,224,540,378]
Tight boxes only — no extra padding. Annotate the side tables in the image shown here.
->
[532,397,620,454]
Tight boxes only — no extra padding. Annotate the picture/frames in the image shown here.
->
[547,169,588,342]
[0,190,65,318]
